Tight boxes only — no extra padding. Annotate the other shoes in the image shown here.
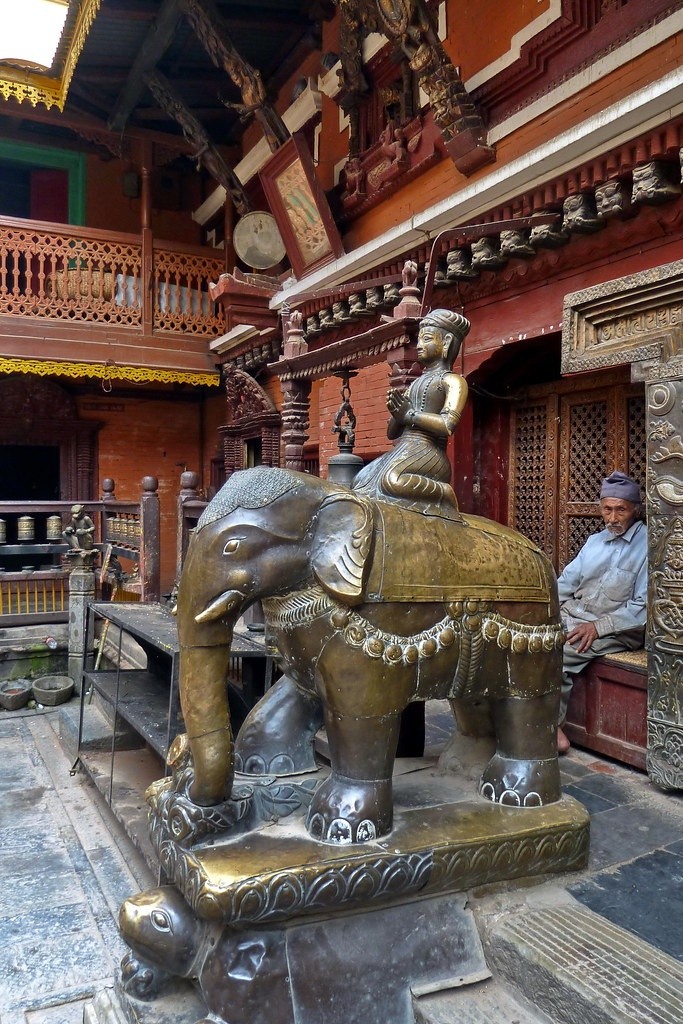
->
[557,727,570,751]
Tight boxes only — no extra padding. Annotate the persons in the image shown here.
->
[174,55,283,213]
[558,472,648,755]
[351,309,471,524]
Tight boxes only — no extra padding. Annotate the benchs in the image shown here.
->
[562,648,647,770]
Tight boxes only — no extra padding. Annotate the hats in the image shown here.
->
[600,470,641,503]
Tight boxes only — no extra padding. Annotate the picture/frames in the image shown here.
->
[258,132,346,282]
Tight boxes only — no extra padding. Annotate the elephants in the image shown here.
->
[170,465,567,846]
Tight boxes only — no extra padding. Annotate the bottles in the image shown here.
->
[46,637,57,649]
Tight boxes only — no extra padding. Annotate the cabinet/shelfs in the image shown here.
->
[68,599,273,885]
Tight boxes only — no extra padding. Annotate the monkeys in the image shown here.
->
[62,504,96,553]
